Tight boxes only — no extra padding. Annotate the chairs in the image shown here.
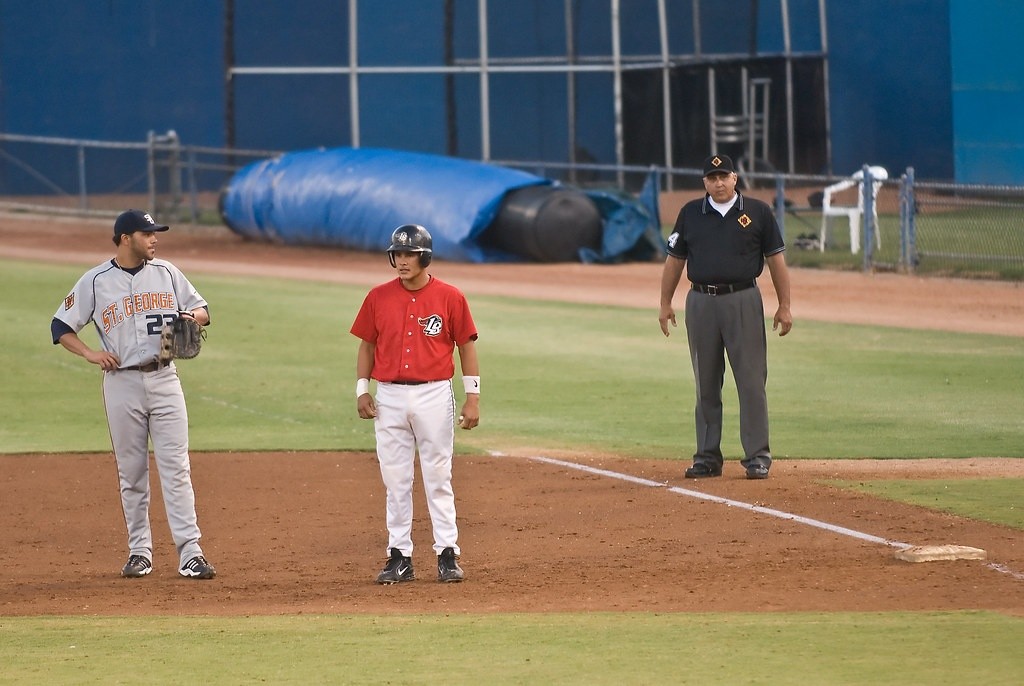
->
[820,165,888,254]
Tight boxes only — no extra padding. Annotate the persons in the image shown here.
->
[349,225,480,585]
[658,155,794,479]
[51,209,217,581]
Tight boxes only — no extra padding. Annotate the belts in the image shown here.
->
[391,381,427,385]
[123,359,170,372]
[691,281,755,296]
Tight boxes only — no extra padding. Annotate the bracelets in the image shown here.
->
[462,374,480,396]
[355,378,370,399]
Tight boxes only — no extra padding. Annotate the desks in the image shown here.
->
[767,206,833,247]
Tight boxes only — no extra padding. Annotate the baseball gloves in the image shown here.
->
[159,314,208,359]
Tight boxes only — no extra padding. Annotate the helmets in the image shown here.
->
[387,224,432,267]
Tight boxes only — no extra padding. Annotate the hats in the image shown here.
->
[113,210,169,235]
[702,155,734,177]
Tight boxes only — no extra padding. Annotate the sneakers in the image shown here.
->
[685,464,722,478]
[437,547,464,582]
[377,548,415,581]
[746,464,768,478]
[178,556,216,578]
[120,555,153,576]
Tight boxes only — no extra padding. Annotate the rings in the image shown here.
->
[459,416,465,421]
